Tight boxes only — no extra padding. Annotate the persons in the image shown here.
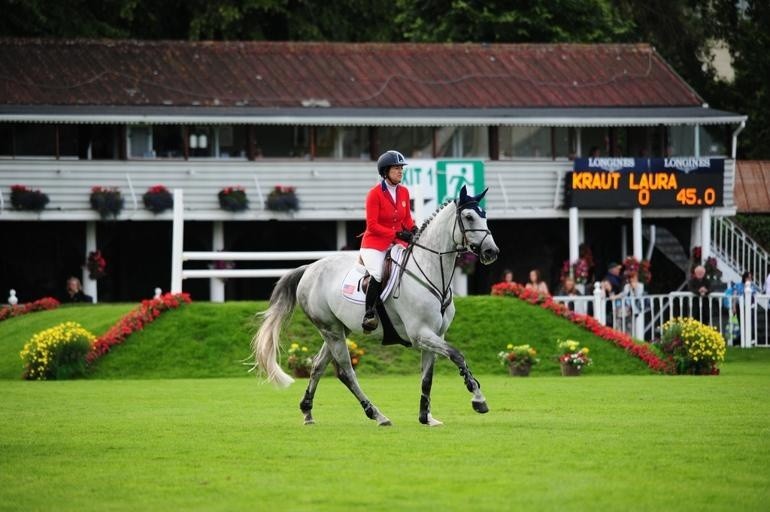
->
[59,277,93,306]
[588,145,602,158]
[358,151,420,335]
[490,262,769,346]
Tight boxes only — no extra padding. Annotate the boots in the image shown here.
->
[364,275,383,329]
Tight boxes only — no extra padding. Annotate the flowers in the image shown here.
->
[285,344,312,369]
[331,339,364,365]
[496,343,541,369]
[555,335,595,369]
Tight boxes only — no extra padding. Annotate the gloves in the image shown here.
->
[398,226,419,242]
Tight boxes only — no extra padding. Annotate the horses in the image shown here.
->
[238,185,501,427]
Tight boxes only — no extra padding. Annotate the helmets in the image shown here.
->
[378,151,408,179]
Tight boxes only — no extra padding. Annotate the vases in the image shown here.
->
[294,366,306,379]
[561,363,581,378]
[508,364,533,377]
[335,362,356,385]
